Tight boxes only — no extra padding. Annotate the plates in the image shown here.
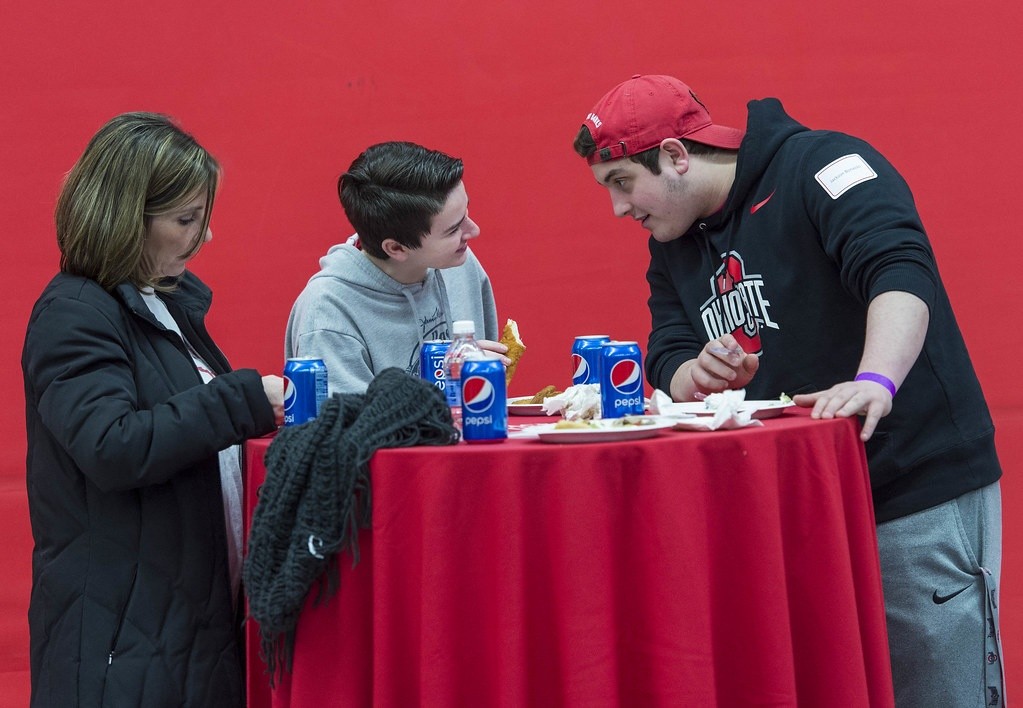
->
[536,415,678,443]
[672,397,796,419]
[506,394,567,416]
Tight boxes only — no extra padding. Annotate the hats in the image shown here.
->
[581,74,747,167]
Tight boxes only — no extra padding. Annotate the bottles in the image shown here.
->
[444,320,486,442]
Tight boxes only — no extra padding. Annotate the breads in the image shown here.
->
[500,318,527,387]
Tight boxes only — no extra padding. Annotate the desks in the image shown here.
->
[243,405,893,707]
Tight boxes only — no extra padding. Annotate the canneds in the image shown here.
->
[570,334,610,384]
[283,356,328,425]
[419,338,453,396]
[599,339,646,419]
[459,356,508,443]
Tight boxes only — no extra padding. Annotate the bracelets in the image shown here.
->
[854,373,896,399]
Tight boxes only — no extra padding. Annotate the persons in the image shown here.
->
[573,73,1006,708]
[20,112,284,708]
[285,141,512,395]
